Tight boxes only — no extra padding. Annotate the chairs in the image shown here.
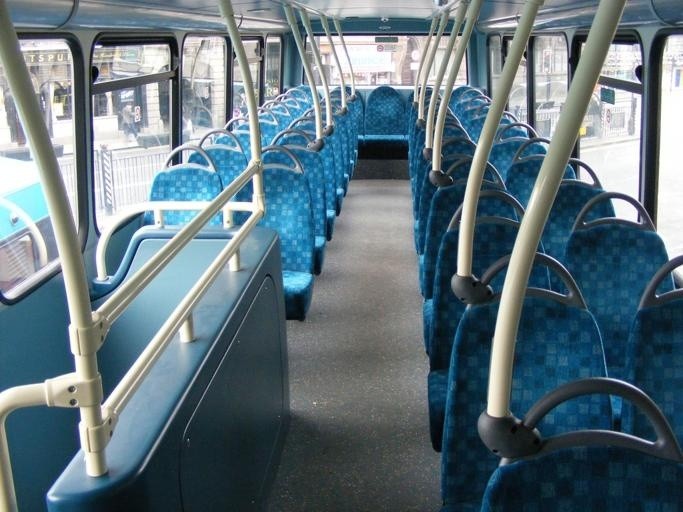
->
[281,88,311,112]
[441,250,615,512]
[421,157,518,359]
[403,88,432,143]
[278,117,336,241]
[143,144,223,226]
[414,137,494,257]
[410,98,440,159]
[472,110,527,155]
[460,95,490,125]
[322,90,358,167]
[428,190,552,453]
[410,113,464,194]
[481,376,682,511]
[412,123,474,219]
[212,117,267,167]
[258,99,293,140]
[289,84,319,106]
[270,94,304,123]
[319,98,354,181]
[234,145,316,322]
[309,104,349,197]
[452,89,487,125]
[408,104,451,174]
[506,136,577,223]
[333,87,364,143]
[555,192,678,423]
[364,84,405,142]
[465,103,513,142]
[493,122,547,185]
[448,86,481,113]
[297,108,344,216]
[621,255,682,446]
[187,129,253,225]
[235,107,281,152]
[261,128,326,275]
[528,156,615,292]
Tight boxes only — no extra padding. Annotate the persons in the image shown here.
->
[121,102,139,143]
